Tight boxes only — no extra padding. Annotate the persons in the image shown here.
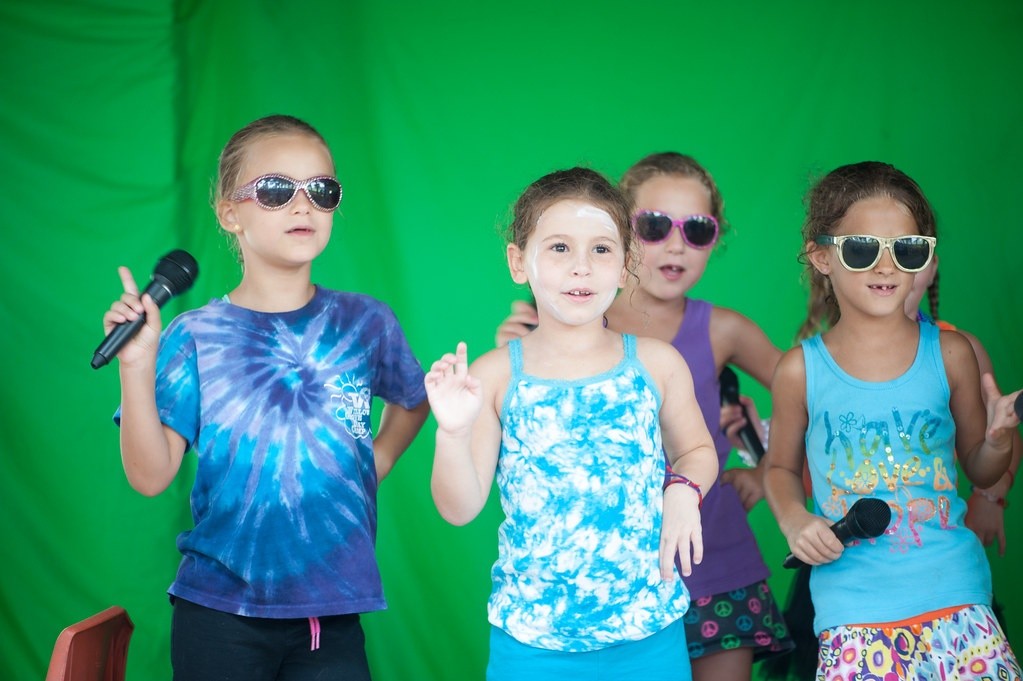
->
[718,159,1022,681]
[103,112,433,680]
[493,149,807,681]
[424,163,720,681]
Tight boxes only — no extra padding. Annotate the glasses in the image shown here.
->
[815,233,937,272]
[230,174,342,212]
[632,209,719,250]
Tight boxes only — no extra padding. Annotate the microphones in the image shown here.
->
[783,498,891,569]
[91,248,199,369]
[717,367,765,466]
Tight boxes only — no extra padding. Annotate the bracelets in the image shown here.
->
[658,466,703,510]
[972,485,1008,509]
[1005,469,1014,485]
